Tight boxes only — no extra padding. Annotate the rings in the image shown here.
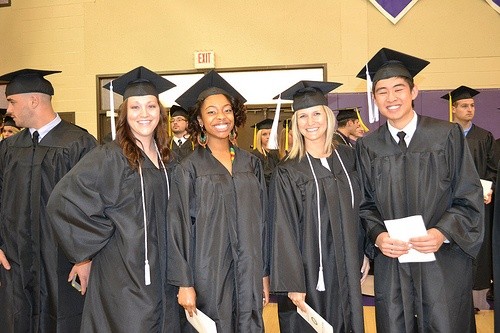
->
[391,249,392,254]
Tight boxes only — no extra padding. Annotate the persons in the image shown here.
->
[0,48,500,333]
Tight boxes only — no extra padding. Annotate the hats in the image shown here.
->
[440,85,481,121]
[356,47,431,124]
[103,65,178,141]
[250,118,281,150]
[265,80,343,149]
[332,106,369,133]
[168,105,189,137]
[0,68,63,97]
[0,115,21,131]
[283,119,293,152]
[175,69,247,116]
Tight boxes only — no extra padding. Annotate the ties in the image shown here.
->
[178,140,182,147]
[397,131,406,150]
[31,131,39,145]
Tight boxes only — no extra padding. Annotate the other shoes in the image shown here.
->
[473,307,480,312]
